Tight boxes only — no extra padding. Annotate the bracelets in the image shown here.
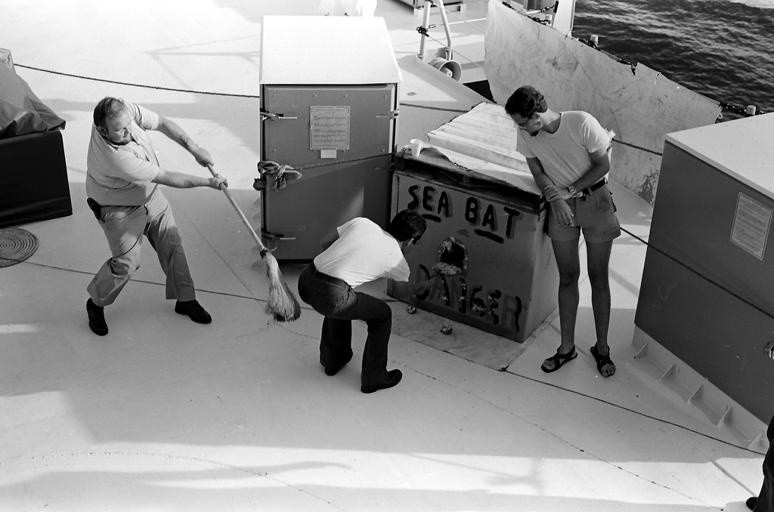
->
[567,185,577,195]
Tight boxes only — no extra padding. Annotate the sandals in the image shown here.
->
[591,342,616,376]
[541,345,578,373]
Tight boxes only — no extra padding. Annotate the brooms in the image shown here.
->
[206,163,301,321]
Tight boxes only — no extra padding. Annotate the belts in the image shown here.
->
[572,178,607,198]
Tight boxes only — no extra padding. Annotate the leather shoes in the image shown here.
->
[87,298,109,335]
[325,351,352,375]
[175,299,211,324]
[361,369,402,393]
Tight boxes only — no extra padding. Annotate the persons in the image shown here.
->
[86,97,228,335]
[298,210,427,394]
[505,85,621,377]
